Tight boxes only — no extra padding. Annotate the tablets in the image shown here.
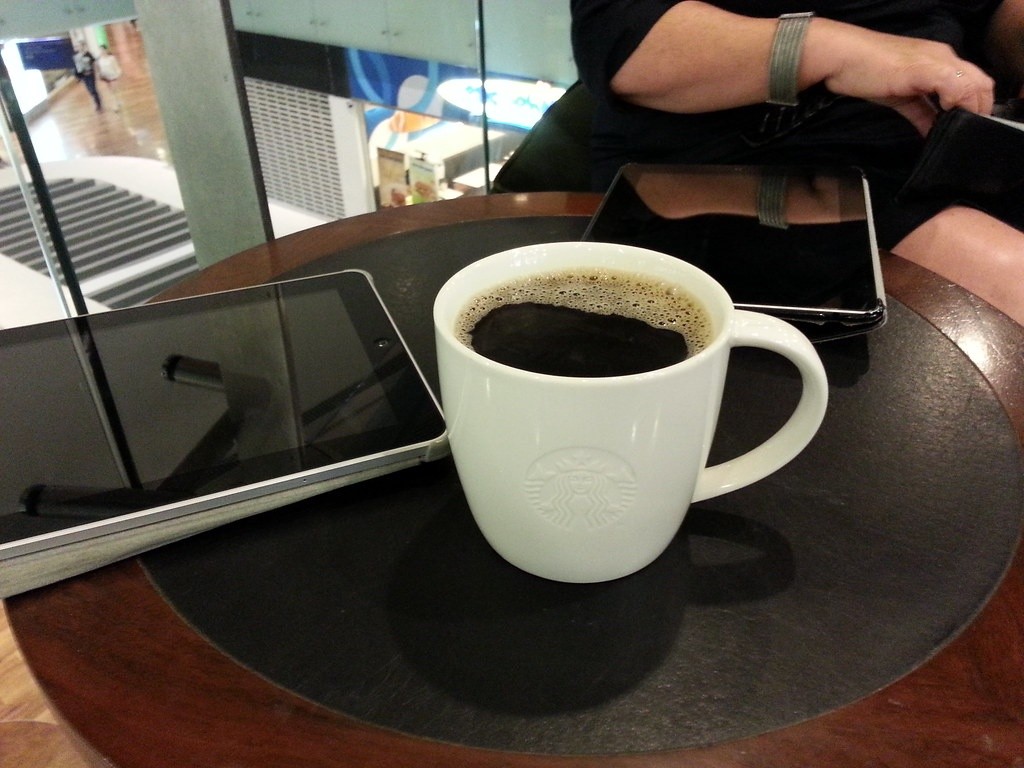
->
[581,162,883,328]
[0,269,447,563]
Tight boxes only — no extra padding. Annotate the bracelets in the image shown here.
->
[756,175,789,230]
[767,11,813,107]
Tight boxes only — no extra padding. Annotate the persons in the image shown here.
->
[74,41,101,111]
[621,164,868,225]
[95,45,121,112]
[569,0,1024,328]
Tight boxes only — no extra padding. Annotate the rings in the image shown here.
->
[956,70,964,78]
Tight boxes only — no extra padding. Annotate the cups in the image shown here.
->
[431,242,829,583]
